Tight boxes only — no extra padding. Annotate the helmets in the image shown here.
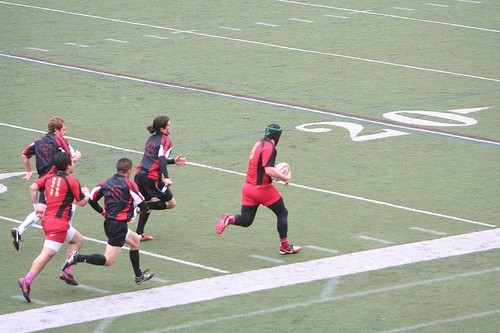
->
[264,123,283,145]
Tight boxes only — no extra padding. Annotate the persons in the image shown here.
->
[61,157,154,284]
[132,116,188,240]
[216,123,301,254]
[10,116,81,250]
[18,152,83,303]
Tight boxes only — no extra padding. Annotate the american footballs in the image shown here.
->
[272,163,289,181]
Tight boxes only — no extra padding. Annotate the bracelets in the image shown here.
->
[33,203,40,209]
[84,193,90,197]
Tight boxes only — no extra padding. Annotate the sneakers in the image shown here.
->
[59,270,79,286]
[215,213,230,233]
[18,277,32,303]
[61,250,79,271]
[279,245,301,254]
[128,206,140,222]
[135,273,155,285]
[9,226,21,251]
[138,233,154,240]
